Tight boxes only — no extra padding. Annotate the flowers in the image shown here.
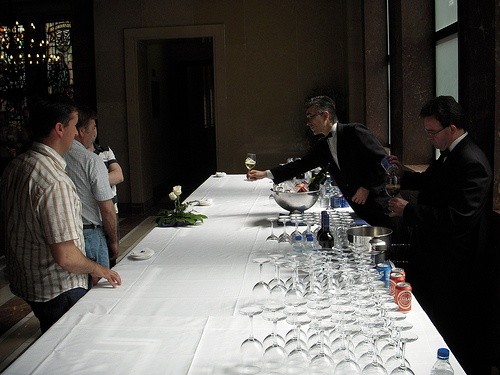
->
[154,185,208,227]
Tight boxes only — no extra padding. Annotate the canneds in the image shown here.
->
[389,268,405,296]
[395,282,412,313]
[325,175,349,209]
[381,156,399,175]
[377,263,391,288]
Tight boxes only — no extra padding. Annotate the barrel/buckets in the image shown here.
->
[346,226,393,264]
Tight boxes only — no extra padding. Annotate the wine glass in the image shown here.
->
[385,175,401,215]
[226,209,417,375]
[245,154,256,181]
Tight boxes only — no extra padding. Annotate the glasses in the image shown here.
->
[423,122,453,137]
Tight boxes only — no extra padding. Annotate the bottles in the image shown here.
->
[430,348,455,375]
[319,214,334,248]
[297,166,349,209]
[316,211,327,243]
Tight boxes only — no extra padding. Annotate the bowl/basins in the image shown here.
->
[269,188,321,216]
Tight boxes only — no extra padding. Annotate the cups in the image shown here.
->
[286,157,302,162]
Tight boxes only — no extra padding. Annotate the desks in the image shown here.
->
[1,172,468,374]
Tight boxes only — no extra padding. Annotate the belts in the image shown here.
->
[83,224,100,230]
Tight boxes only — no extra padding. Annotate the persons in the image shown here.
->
[246,95,403,245]
[88,127,123,215]
[388,96,492,340]
[0,98,121,334]
[64,108,119,285]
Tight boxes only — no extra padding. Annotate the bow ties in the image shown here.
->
[440,147,451,158]
[319,131,332,142]
